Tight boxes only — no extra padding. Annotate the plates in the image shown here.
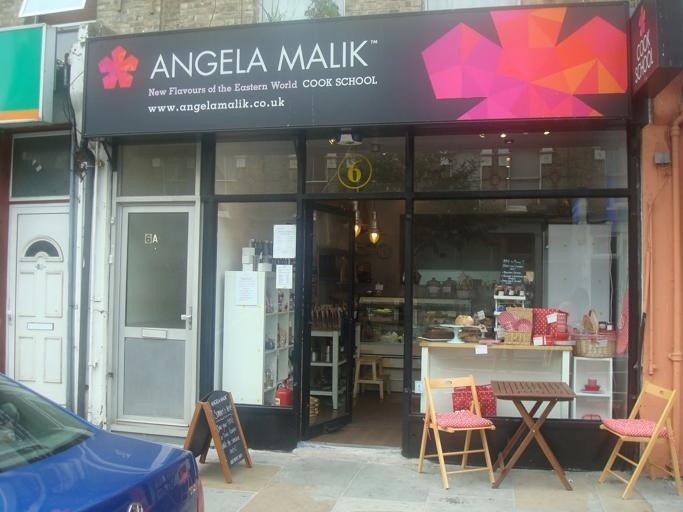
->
[418,336,450,342]
[371,310,394,315]
[580,390,603,394]
[554,340,577,346]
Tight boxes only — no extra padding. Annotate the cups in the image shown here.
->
[588,379,597,385]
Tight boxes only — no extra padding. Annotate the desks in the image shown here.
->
[418,338,572,418]
[489,378,575,491]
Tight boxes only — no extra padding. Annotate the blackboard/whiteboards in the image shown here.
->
[185,391,244,470]
[499,257,527,284]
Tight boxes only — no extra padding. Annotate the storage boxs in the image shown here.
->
[533,307,568,344]
[504,306,533,345]
[451,385,497,417]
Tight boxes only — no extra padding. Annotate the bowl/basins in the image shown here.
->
[554,332,569,340]
[585,385,600,390]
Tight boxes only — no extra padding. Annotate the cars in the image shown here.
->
[0,373,203,512]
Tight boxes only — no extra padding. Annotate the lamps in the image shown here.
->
[368,199,383,244]
[352,201,362,237]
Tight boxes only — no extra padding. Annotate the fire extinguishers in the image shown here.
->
[274,378,292,407]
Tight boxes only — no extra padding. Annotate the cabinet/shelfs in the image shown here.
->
[358,297,472,393]
[222,270,297,407]
[492,287,526,340]
[310,324,363,409]
[572,357,614,421]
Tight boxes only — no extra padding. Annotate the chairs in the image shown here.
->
[418,376,495,489]
[598,382,683,499]
